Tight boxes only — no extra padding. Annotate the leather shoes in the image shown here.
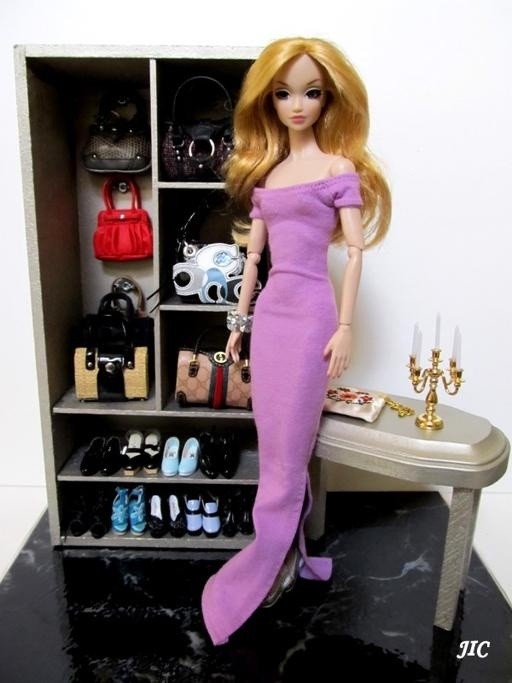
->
[80,430,234,479]
[70,485,253,538]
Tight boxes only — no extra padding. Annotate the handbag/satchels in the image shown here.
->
[175,317,251,411]
[73,76,155,400]
[162,76,233,183]
